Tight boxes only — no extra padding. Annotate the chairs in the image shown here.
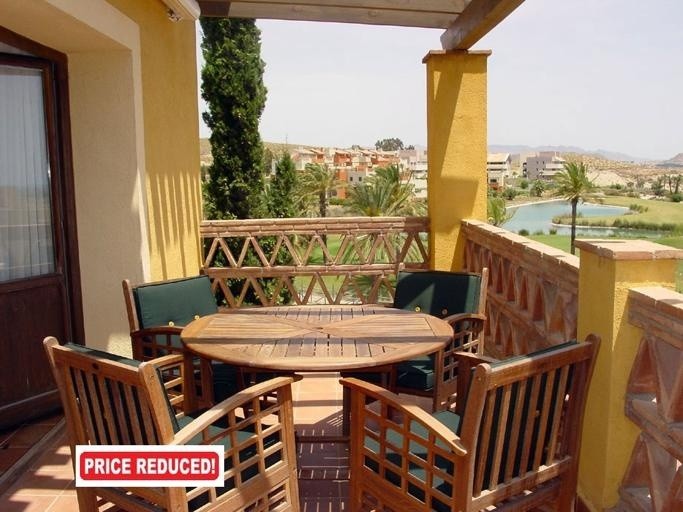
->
[43,262,601,512]
